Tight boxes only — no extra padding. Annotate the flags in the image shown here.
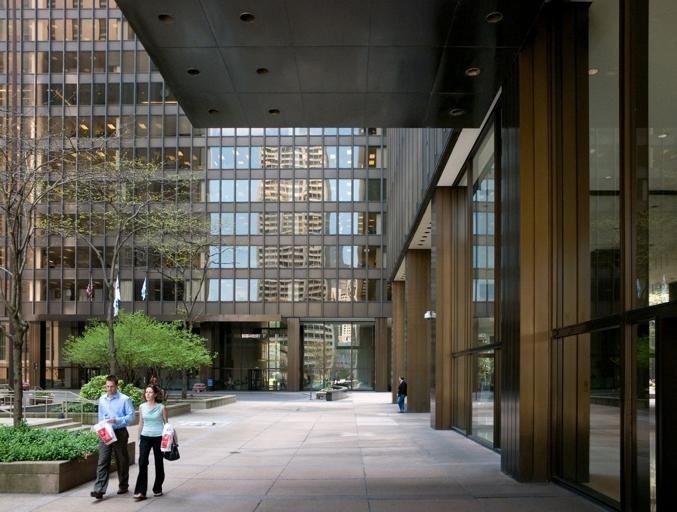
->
[113,275,121,317]
[87,274,95,297]
[141,276,147,301]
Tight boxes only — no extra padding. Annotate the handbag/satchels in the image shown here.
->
[164,445,179,460]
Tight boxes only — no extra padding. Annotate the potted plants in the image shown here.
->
[28,390,54,405]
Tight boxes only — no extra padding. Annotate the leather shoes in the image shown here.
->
[133,493,144,499]
[153,492,162,496]
[117,489,128,493]
[91,492,102,499]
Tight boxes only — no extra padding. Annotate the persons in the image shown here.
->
[134,384,168,501]
[90,375,136,499]
[397,376,407,413]
[150,375,165,403]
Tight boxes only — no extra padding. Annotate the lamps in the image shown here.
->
[424,310,437,320]
